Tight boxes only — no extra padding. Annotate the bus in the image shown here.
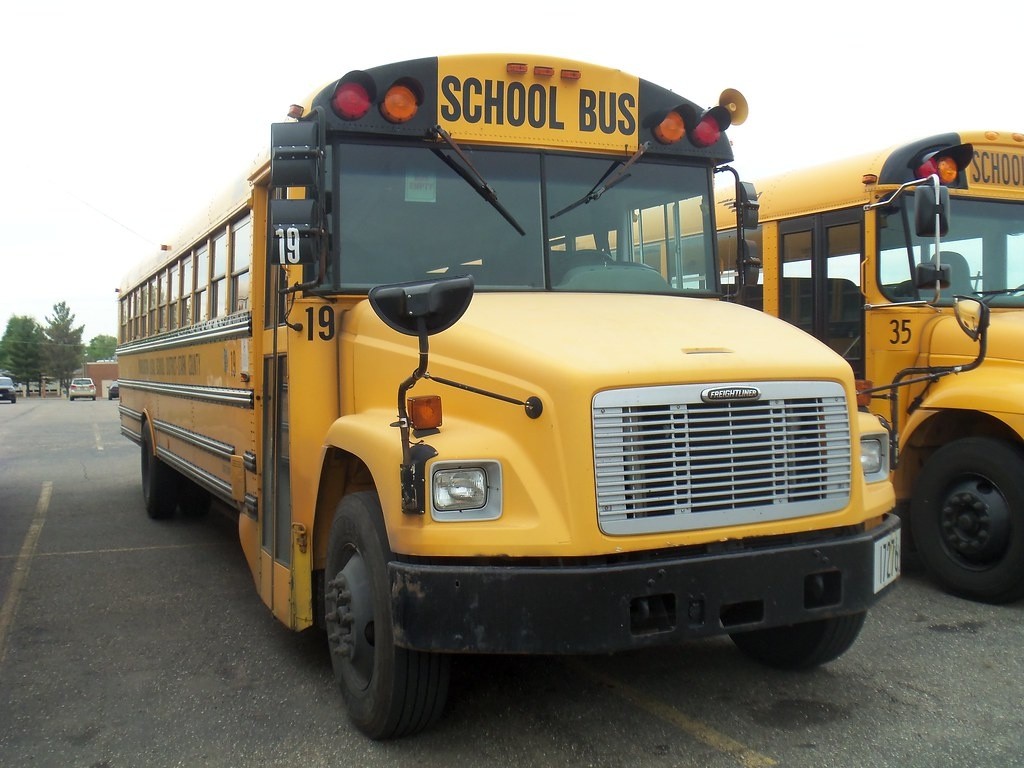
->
[516,129,1024,602]
[117,50,910,738]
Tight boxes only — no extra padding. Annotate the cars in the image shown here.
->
[0,376,16,403]
[69,378,97,400]
[106,381,122,401]
